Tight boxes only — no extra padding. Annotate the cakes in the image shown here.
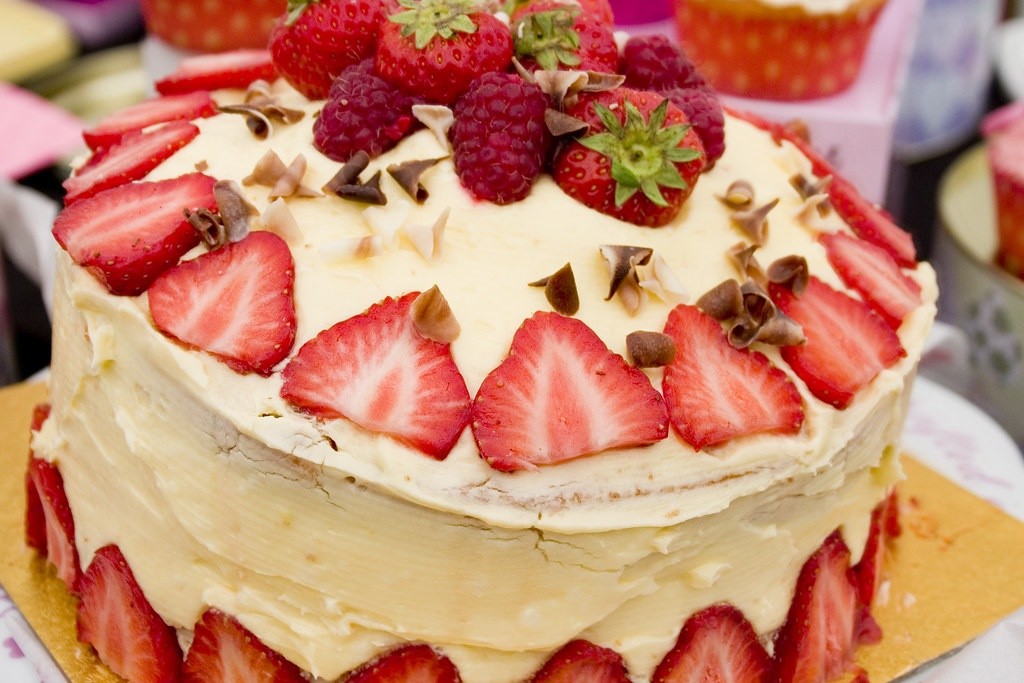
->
[21,0,940,683]
